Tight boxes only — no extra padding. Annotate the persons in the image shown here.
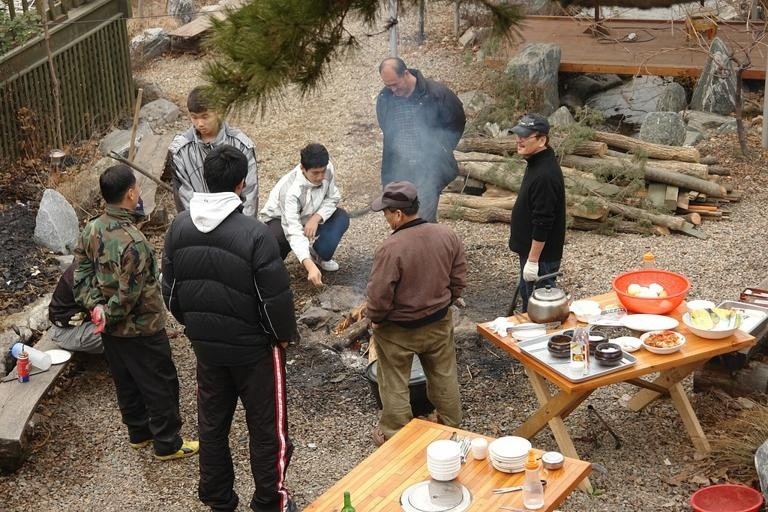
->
[72,166,201,461]
[259,143,350,289]
[161,143,297,512]
[507,113,566,314]
[167,85,258,218]
[376,57,466,224]
[364,180,467,448]
[47,255,105,355]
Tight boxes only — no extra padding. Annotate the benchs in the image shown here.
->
[167,8,229,60]
[0,329,75,471]
[126,131,176,227]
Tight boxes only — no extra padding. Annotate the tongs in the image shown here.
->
[505,320,561,335]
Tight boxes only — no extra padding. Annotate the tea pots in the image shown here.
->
[526,271,576,329]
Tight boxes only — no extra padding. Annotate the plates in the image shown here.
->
[43,349,71,365]
[426,439,462,483]
[488,435,534,475]
[512,300,687,357]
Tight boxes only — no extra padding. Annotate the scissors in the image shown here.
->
[492,480,547,494]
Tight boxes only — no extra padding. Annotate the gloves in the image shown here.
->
[90,303,106,335]
[522,258,539,283]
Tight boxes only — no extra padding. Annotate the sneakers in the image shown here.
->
[153,439,199,461]
[310,250,340,272]
[129,438,154,449]
[373,429,389,447]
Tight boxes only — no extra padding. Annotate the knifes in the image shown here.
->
[451,430,473,466]
[498,505,534,512]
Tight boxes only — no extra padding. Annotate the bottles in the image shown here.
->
[522,446,547,510]
[641,247,658,271]
[17,342,30,383]
[569,324,591,379]
[11,341,52,372]
[338,491,356,512]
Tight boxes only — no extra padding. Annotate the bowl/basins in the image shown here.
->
[541,451,565,471]
[682,308,745,339]
[686,299,716,310]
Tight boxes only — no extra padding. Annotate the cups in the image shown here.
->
[595,343,622,366]
[469,436,488,462]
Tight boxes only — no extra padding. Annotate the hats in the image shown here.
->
[371,180,418,212]
[507,113,550,139]
